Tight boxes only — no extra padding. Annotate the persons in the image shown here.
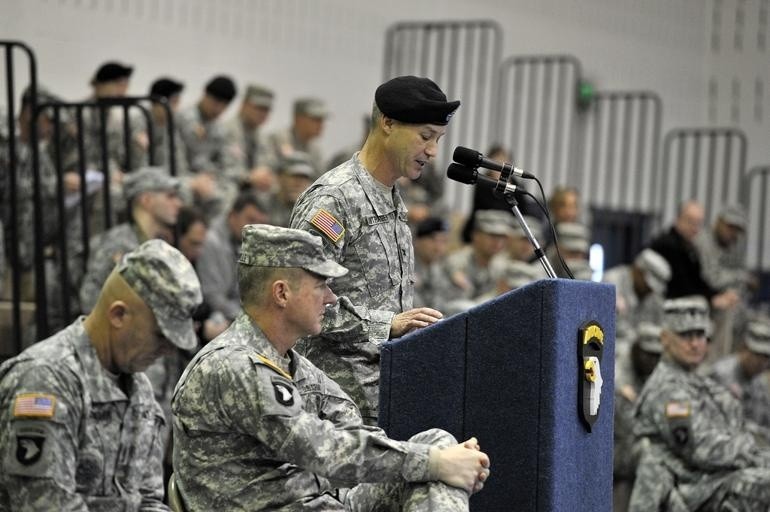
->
[0,239,203,512]
[148,76,206,177]
[695,200,759,292]
[270,94,331,177]
[289,75,461,429]
[196,195,276,320]
[549,184,582,222]
[266,152,317,226]
[627,295,770,512]
[182,75,247,176]
[1,86,105,268]
[489,217,543,284]
[463,145,527,242]
[614,323,666,435]
[175,210,210,260]
[223,82,281,202]
[410,217,473,318]
[536,223,594,280]
[73,62,177,236]
[79,166,182,317]
[707,320,770,432]
[450,209,513,309]
[601,246,674,336]
[170,223,491,512]
[640,200,720,302]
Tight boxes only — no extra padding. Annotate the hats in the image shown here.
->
[719,205,752,233]
[91,63,133,86]
[118,239,204,351]
[24,87,70,123]
[661,296,711,334]
[375,76,461,126]
[294,99,332,119]
[416,217,448,238]
[237,225,350,278]
[206,77,236,103]
[404,186,431,205]
[635,249,672,294]
[556,223,590,254]
[148,80,183,104]
[516,216,543,240]
[743,322,770,354]
[474,210,515,239]
[283,151,315,179]
[122,167,183,199]
[247,86,274,109]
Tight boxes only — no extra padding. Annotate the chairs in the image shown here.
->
[167,473,186,512]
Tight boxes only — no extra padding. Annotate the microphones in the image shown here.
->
[452,146,540,179]
[445,162,532,195]
